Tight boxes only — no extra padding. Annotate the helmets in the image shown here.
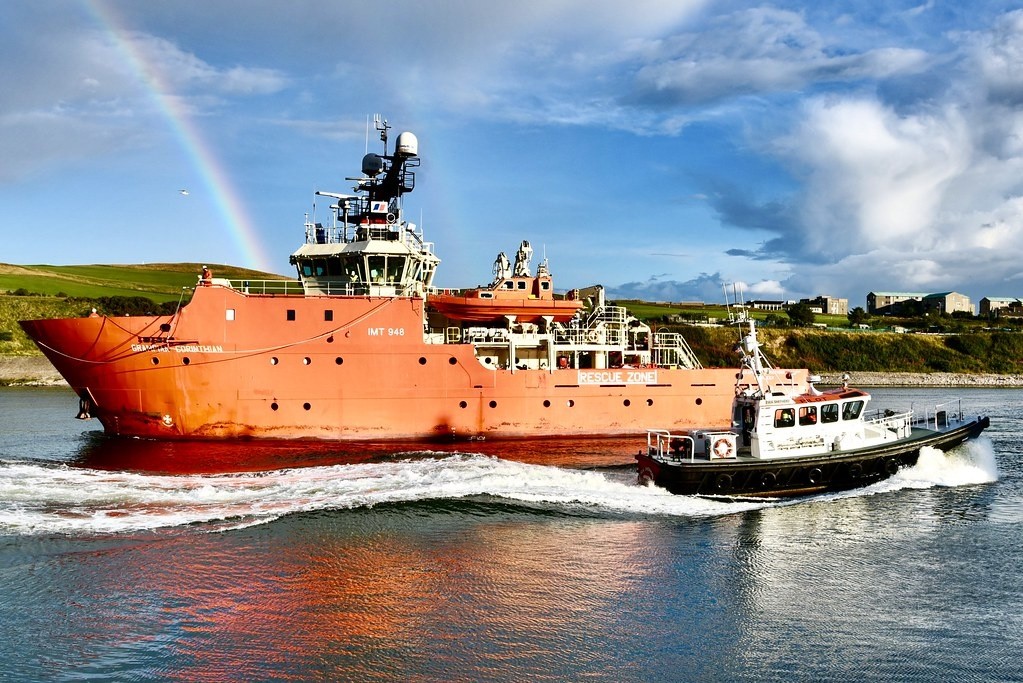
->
[203,265,207,270]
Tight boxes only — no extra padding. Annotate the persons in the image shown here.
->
[202,265,212,287]
[884,409,895,417]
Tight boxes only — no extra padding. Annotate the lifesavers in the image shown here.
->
[886,460,899,475]
[716,473,732,490]
[589,331,599,341]
[762,472,776,488]
[714,439,732,457]
[848,463,863,477]
[809,467,822,482]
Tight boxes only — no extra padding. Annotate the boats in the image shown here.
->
[633,282,992,501]
[18,114,869,443]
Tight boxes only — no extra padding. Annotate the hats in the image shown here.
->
[351,271,355,276]
